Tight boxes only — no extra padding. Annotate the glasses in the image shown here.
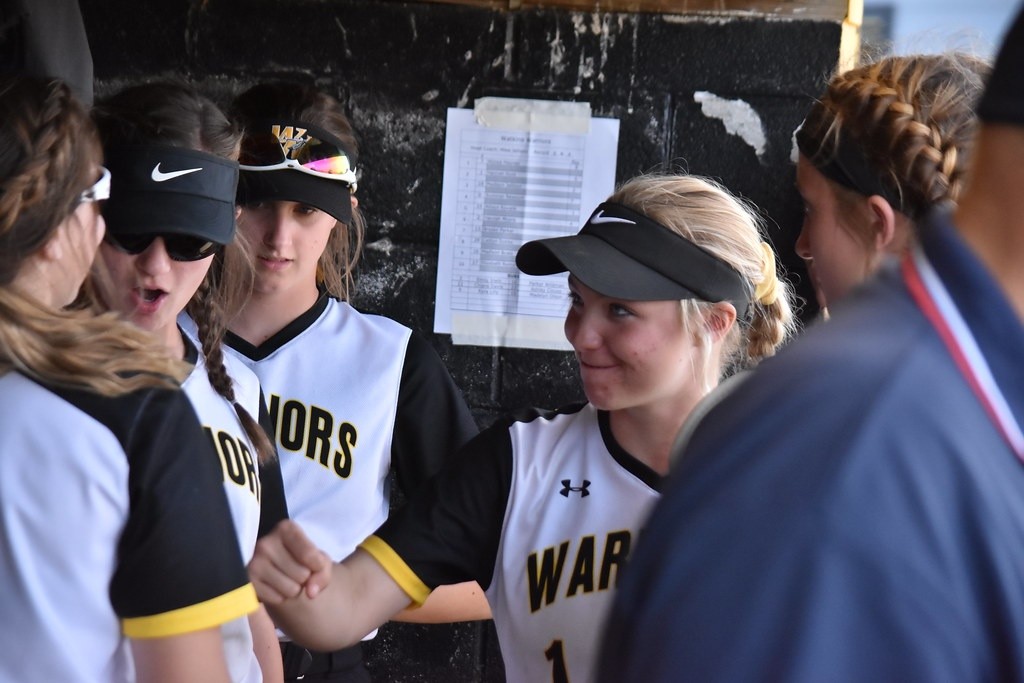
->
[237,131,357,194]
[81,166,110,201]
[104,228,221,261]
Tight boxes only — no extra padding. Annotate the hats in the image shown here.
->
[235,122,353,225]
[514,201,754,326]
[103,142,238,245]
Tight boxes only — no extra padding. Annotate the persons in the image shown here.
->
[792,52,998,321]
[174,82,494,683]
[247,173,791,683]
[600,4,1024,683]
[0,72,287,683]
